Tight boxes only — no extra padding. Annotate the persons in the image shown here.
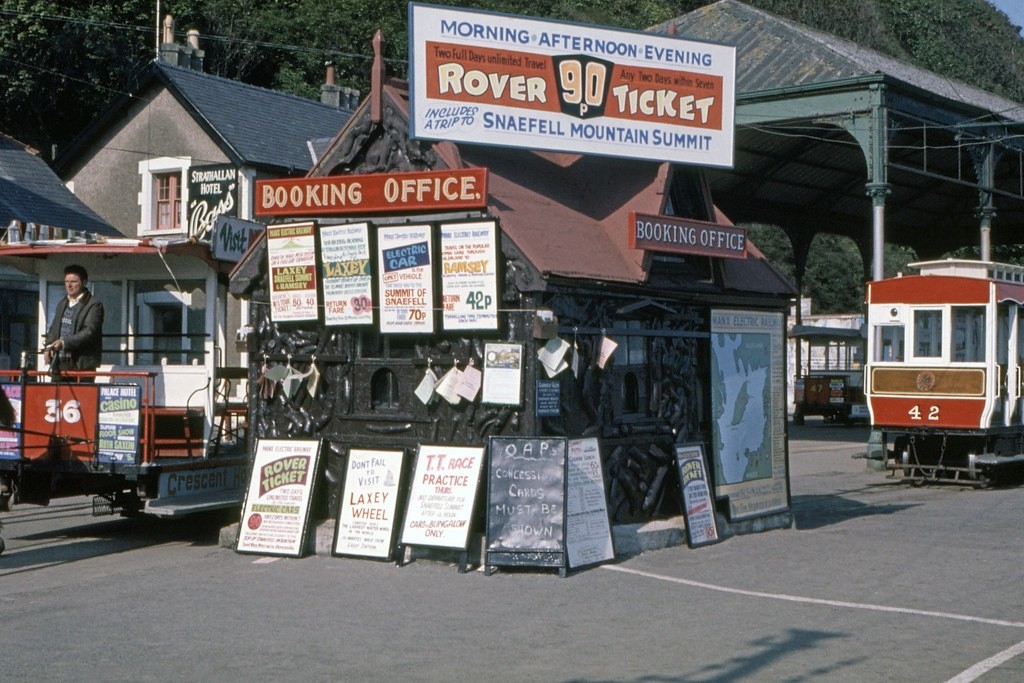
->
[42,265,105,384]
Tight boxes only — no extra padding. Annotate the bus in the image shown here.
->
[857,257,1024,489]
[0,222,263,519]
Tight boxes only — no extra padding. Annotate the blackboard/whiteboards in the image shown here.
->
[484,435,569,567]
[536,377,561,417]
